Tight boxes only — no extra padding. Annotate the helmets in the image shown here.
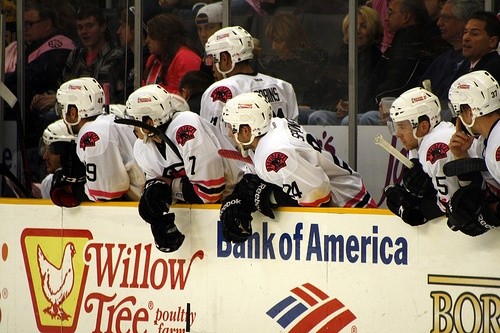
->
[170,93,190,112]
[108,104,127,119]
[390,87,442,127]
[205,25,255,64]
[126,84,173,126]
[448,70,500,117]
[55,77,105,118]
[42,119,78,145]
[222,93,272,137]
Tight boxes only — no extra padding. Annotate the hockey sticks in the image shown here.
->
[113,118,183,163]
[217,149,254,166]
[373,133,414,170]
[0,163,34,197]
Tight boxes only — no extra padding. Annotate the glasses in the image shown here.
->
[25,19,48,28]
[440,14,456,22]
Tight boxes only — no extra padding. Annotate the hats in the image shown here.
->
[195,2,223,24]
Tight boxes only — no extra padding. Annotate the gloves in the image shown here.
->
[220,179,253,244]
[151,212,185,253]
[243,173,282,219]
[138,179,173,223]
[50,150,88,208]
[402,158,438,196]
[383,184,429,227]
[445,177,500,237]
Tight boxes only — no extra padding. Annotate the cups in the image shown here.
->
[380,97,395,124]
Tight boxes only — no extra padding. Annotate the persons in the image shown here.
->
[224,91,379,243]
[121,83,256,224]
[447,70,500,237]
[31,120,81,201]
[199,24,300,154]
[0,0,500,157]
[387,86,478,227]
[50,75,146,203]
